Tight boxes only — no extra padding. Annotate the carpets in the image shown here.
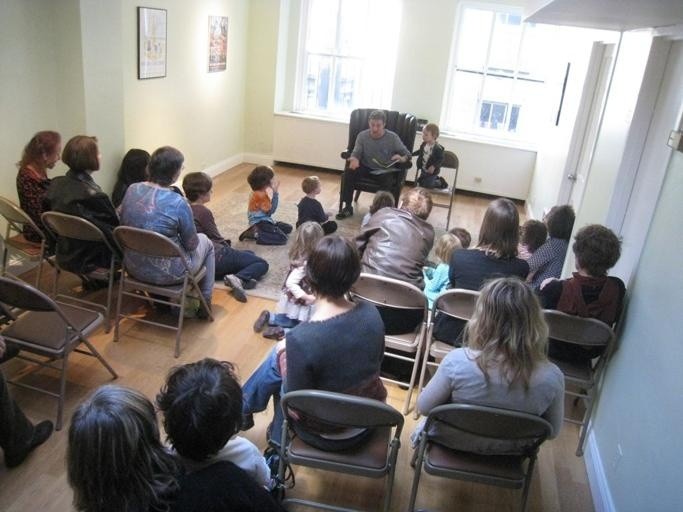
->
[207,192,481,302]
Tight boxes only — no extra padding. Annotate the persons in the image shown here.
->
[431,228,471,269]
[238,165,292,245]
[356,190,396,259]
[112,148,182,216]
[1,338,52,466]
[66,387,281,511]
[48,135,126,292]
[412,123,449,189]
[118,146,216,319]
[156,357,274,492]
[424,234,463,320]
[537,223,628,369]
[517,219,547,285]
[337,111,413,220]
[347,187,434,387]
[254,222,326,338]
[235,236,387,476]
[411,277,567,468]
[419,198,529,373]
[525,205,576,296]
[297,177,337,238]
[183,172,268,302]
[16,132,63,273]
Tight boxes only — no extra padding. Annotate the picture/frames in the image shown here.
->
[208,16,228,72]
[136,6,167,80]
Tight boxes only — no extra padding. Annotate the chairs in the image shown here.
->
[415,151,459,231]
[339,108,417,211]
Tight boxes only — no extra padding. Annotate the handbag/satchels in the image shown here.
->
[264,443,295,491]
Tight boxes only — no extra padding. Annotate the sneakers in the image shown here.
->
[440,176,448,188]
[254,311,270,333]
[239,225,255,241]
[151,310,177,325]
[335,207,354,220]
[262,327,285,341]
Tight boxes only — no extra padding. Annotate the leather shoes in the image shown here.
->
[223,278,257,289]
[240,414,254,431]
[224,274,247,302]
[0,344,20,364]
[4,420,53,469]
